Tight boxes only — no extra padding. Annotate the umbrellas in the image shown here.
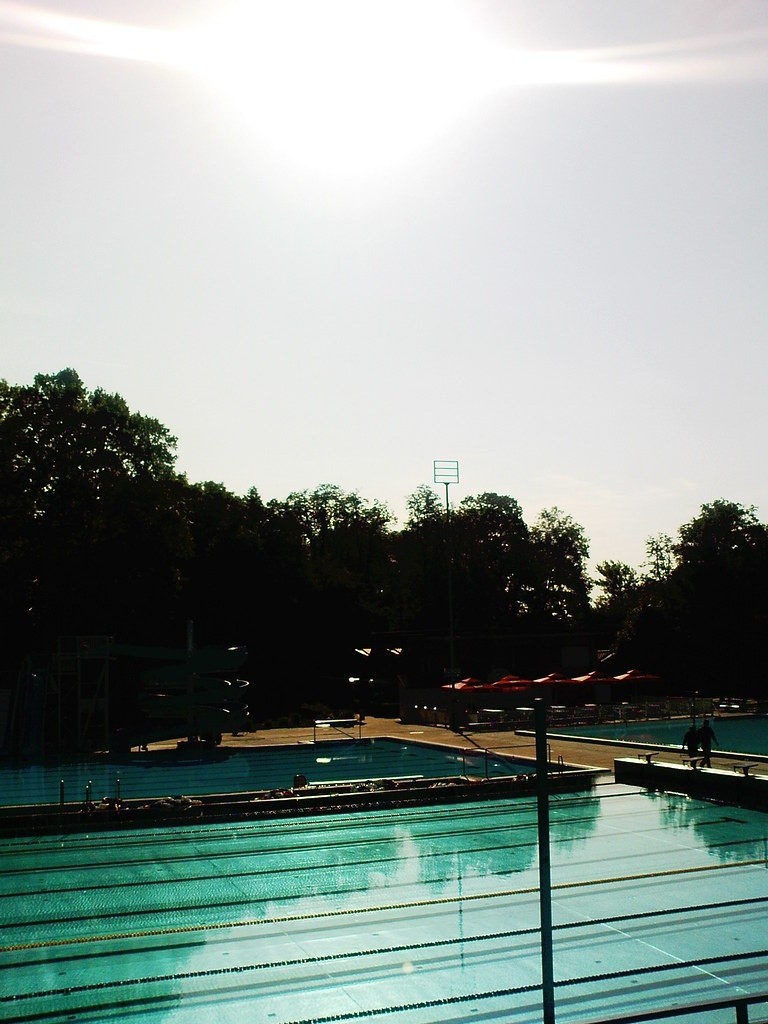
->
[440,668,660,710]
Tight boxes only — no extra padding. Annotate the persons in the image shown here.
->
[682,720,719,770]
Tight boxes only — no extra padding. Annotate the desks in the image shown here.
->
[314,719,361,741]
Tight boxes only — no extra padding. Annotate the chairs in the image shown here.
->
[477,706,671,730]
